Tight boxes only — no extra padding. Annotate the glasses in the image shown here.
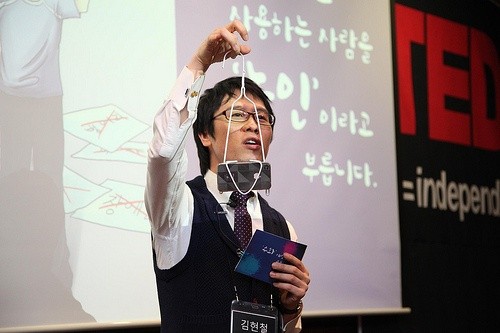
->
[211,109,274,126]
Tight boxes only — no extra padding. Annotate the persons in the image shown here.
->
[142,18,311,331]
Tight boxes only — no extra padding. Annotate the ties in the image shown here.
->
[230,190,254,248]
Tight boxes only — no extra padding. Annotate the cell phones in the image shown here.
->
[217,163,272,191]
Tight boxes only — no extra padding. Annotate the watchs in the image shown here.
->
[279,300,304,315]
